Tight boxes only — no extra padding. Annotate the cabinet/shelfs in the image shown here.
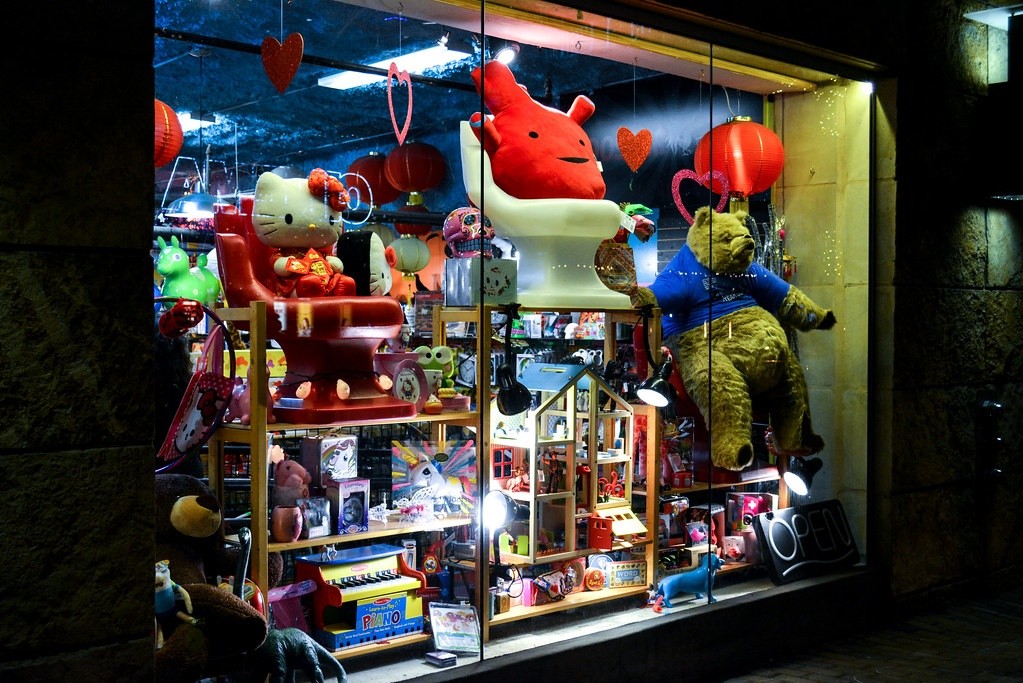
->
[208,302,790,660]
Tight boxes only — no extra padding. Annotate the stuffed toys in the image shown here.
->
[155,473,270,683]
[469,60,607,199]
[271,444,311,507]
[253,166,354,299]
[336,228,398,297]
[630,206,836,472]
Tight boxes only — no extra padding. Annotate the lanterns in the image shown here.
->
[154,100,184,168]
[694,116,785,213]
[347,138,446,309]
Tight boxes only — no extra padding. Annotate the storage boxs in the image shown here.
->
[327,477,370,535]
[444,259,517,305]
[301,434,358,489]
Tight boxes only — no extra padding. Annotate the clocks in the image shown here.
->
[460,360,474,382]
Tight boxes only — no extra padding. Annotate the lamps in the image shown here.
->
[784,456,824,495]
[164,48,230,217]
[496,301,532,416]
[437,25,450,45]
[470,33,520,65]
[634,304,673,407]
